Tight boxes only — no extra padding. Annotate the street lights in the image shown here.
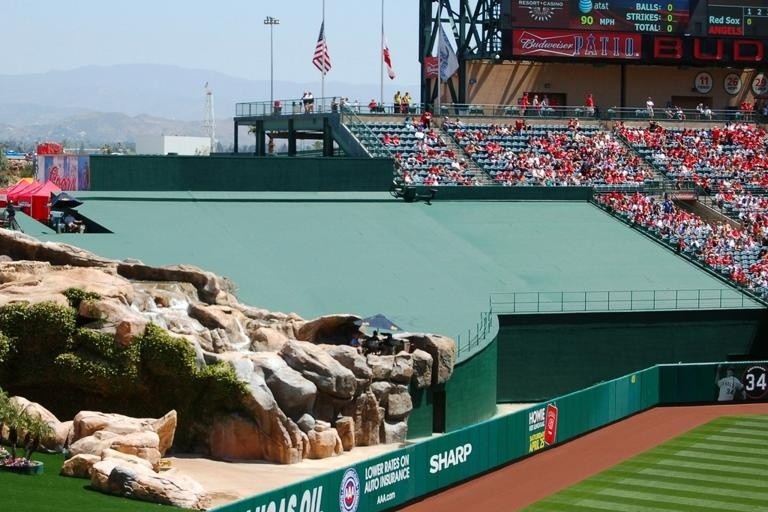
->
[264,16,279,153]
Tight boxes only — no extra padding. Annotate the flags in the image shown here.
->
[437,21,460,85]
[382,19,395,81]
[311,22,332,74]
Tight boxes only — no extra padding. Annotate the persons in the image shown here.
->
[519,91,755,121]
[300,88,412,115]
[595,118,768,302]
[4,200,16,230]
[64,211,86,234]
[382,110,658,185]
[351,330,358,350]
[366,330,380,352]
[714,364,747,404]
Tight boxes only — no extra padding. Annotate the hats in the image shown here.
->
[727,366,735,372]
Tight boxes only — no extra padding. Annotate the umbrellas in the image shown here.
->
[49,191,84,213]
[352,312,403,333]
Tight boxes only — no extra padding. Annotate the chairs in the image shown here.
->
[342,105,586,202]
[586,105,768,306]
